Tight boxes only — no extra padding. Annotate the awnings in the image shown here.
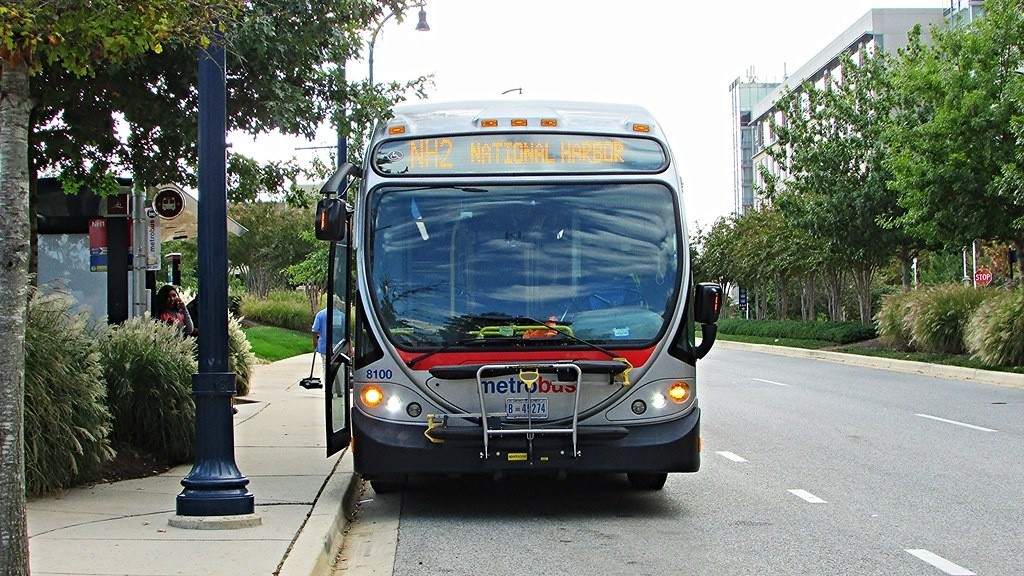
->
[156,181,249,243]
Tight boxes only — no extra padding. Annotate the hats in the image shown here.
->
[333,295,344,306]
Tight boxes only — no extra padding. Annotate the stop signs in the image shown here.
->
[974,269,992,285]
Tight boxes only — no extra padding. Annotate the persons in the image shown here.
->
[157,285,194,335]
[575,248,648,311]
[181,294,198,361]
[312,295,353,397]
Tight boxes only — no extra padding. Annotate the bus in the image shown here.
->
[314,93,723,502]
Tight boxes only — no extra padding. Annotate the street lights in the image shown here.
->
[370,2,429,126]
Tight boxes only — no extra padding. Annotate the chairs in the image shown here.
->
[586,272,641,312]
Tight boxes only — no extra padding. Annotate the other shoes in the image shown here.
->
[337,393,344,398]
